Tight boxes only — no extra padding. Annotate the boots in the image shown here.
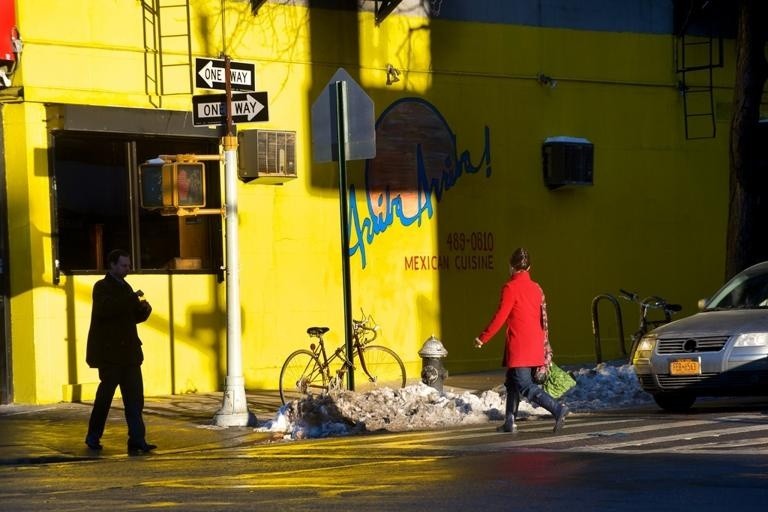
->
[532,388,569,434]
[496,391,520,433]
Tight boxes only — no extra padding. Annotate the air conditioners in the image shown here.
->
[238,128,299,183]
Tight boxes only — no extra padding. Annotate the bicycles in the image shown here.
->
[278,316,407,413]
[619,286,681,368]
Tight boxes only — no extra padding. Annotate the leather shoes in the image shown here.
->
[85,440,103,450]
[128,444,157,454]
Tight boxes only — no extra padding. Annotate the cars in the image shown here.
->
[633,261,768,411]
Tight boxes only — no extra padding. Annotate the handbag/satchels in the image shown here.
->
[532,332,554,386]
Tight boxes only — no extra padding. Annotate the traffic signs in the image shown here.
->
[191,54,271,427]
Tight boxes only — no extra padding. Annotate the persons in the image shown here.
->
[469,246,570,434]
[81,248,158,456]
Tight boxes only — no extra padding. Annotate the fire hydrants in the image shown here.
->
[418,335,448,395]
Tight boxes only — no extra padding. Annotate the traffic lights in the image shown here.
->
[170,161,205,209]
